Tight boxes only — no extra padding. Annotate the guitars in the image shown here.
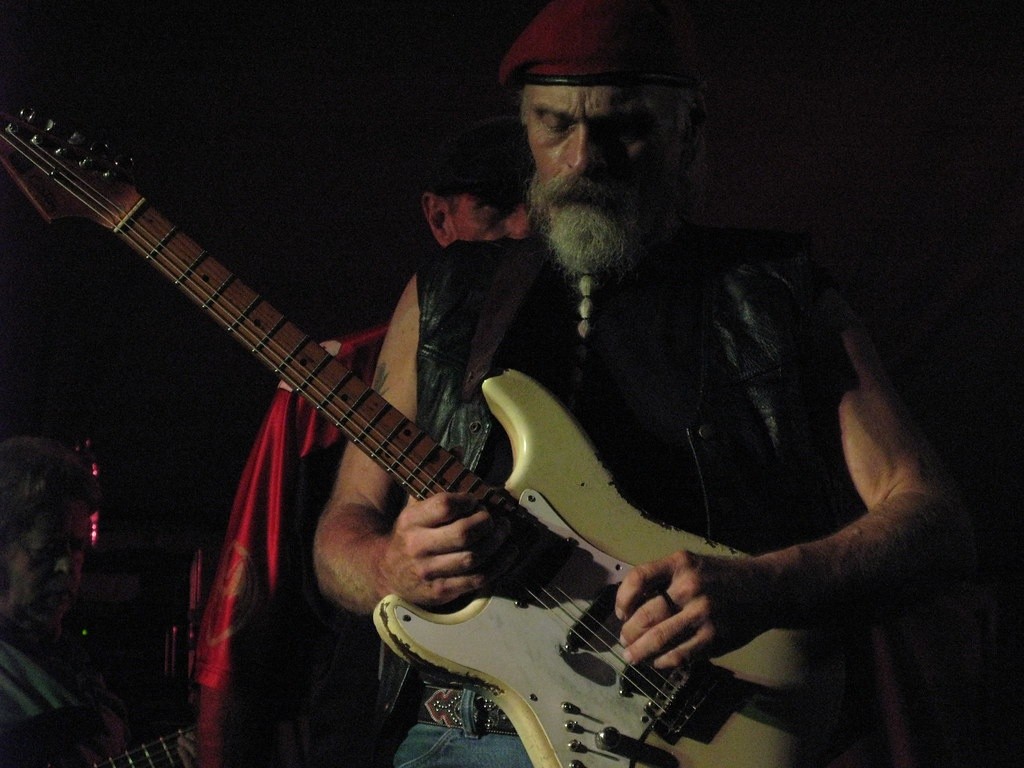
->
[93,723,198,768]
[1,101,847,767]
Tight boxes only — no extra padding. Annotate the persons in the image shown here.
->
[196,112,547,768]
[0,436,132,768]
[311,1,988,768]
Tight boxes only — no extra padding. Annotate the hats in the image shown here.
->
[499,2,701,94]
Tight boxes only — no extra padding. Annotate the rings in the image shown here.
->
[660,587,681,614]
